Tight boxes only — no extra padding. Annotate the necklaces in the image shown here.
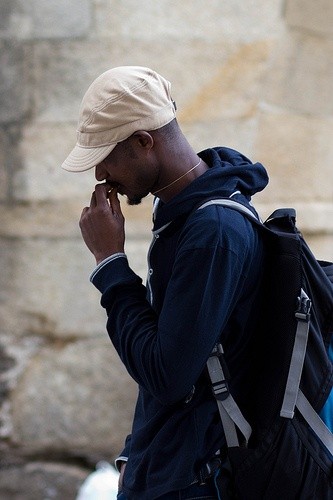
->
[110,158,202,202]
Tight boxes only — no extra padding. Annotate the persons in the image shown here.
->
[60,66,269,500]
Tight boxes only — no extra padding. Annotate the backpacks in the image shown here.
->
[159,198,332,500]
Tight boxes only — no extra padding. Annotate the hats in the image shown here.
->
[60,66,177,173]
